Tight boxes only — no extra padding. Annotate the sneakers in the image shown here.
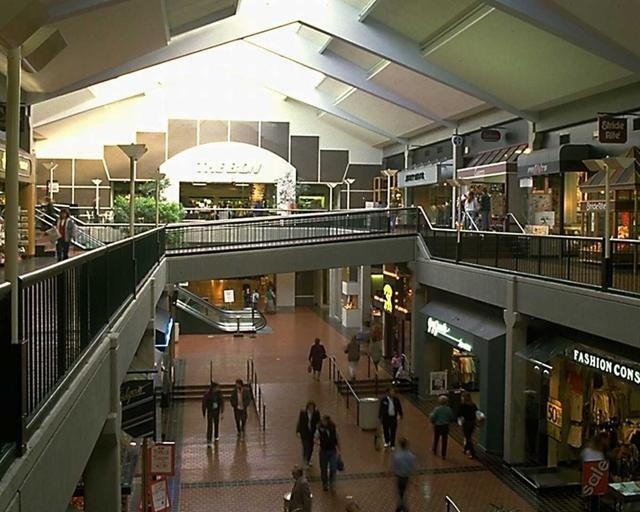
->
[208,444,212,448]
[323,487,328,491]
[215,437,220,441]
[384,442,395,452]
[304,460,312,470]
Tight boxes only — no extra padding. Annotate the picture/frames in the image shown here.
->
[148,478,172,512]
[148,441,176,476]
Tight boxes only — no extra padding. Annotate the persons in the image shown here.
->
[307,337,329,382]
[244,286,250,305]
[463,190,477,229]
[344,335,360,384]
[186,194,269,219]
[264,286,277,314]
[230,377,253,436]
[391,353,401,385]
[55,208,76,263]
[479,188,491,230]
[200,382,225,445]
[388,198,398,233]
[284,387,481,512]
[251,287,260,310]
[40,195,57,226]
[368,336,381,377]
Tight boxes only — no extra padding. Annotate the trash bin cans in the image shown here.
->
[359,398,380,431]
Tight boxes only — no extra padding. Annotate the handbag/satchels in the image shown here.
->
[337,455,344,471]
[374,434,384,451]
